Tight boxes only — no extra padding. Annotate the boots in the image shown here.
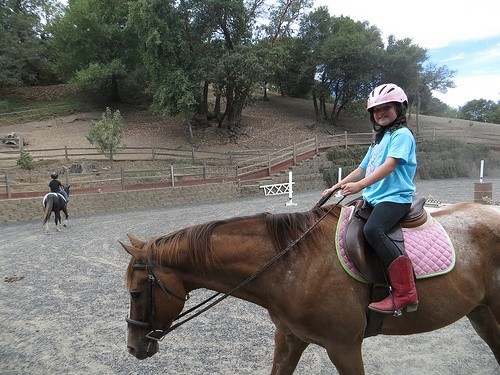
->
[367,255,418,313]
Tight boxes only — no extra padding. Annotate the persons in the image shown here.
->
[39,172,70,203]
[322,84,423,315]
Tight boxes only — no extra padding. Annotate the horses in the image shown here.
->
[38,185,72,234]
[118,201,500,375]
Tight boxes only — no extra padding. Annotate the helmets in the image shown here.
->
[366,82,409,113]
[51,172,58,179]
